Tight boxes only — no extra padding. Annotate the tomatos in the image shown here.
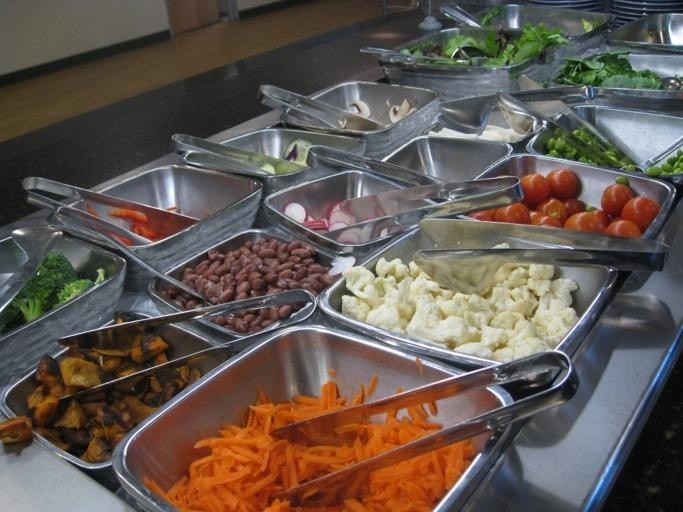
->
[468,169,661,241]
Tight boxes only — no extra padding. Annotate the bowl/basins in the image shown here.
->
[279,80,440,159]
[456,152,679,237]
[1,229,127,386]
[317,221,623,387]
[526,103,682,189]
[47,154,266,288]
[4,311,238,470]
[379,134,514,189]
[148,227,353,338]
[569,52,682,99]
[380,26,564,102]
[604,11,683,53]
[114,326,517,512]
[263,171,440,253]
[460,3,611,48]
[185,126,369,192]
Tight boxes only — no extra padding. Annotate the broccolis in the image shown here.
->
[342,243,579,365]
[14,252,105,324]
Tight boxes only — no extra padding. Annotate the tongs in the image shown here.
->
[329,175,523,243]
[414,219,669,272]
[271,350,578,501]
[496,74,638,170]
[304,143,446,190]
[44,289,320,400]
[170,134,307,175]
[18,174,202,247]
[257,84,386,132]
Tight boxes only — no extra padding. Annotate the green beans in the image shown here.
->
[546,128,683,186]
[163,238,334,334]
